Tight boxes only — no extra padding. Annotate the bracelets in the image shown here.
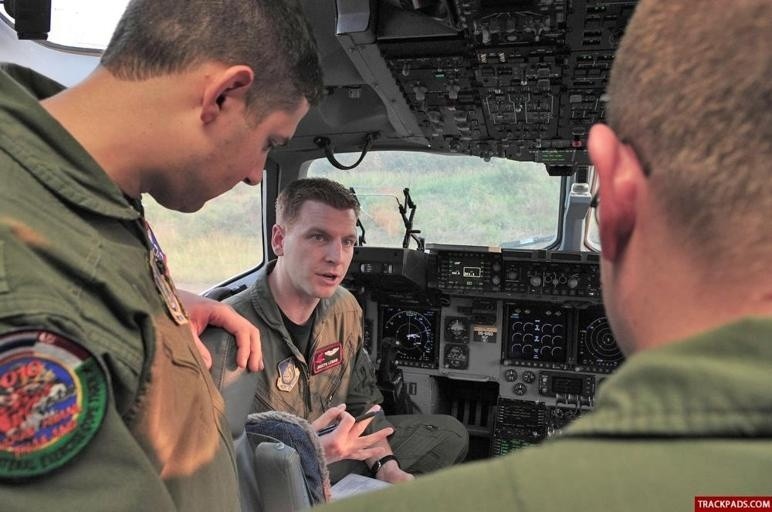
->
[371,454,401,477]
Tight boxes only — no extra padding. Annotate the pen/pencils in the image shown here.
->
[317,411,381,436]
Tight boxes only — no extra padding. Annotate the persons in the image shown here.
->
[219,176,469,486]
[1,1,328,510]
[304,0,768,510]
